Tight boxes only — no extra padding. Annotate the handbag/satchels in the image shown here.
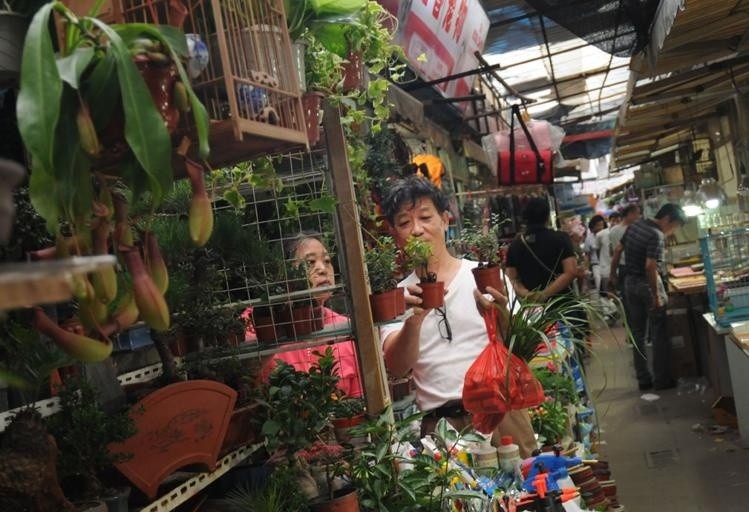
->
[491,104,554,184]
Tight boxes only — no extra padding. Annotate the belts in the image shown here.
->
[423,405,469,419]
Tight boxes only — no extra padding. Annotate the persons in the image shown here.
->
[578,201,655,348]
[234,231,363,462]
[607,200,690,392]
[372,173,541,458]
[502,195,591,342]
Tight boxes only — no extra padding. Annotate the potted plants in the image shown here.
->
[1,3,646,511]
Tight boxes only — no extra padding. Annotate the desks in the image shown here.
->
[664,276,749,446]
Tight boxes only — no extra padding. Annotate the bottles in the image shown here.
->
[497,436,521,474]
[474,440,499,479]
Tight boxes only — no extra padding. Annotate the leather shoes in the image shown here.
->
[639,379,676,391]
